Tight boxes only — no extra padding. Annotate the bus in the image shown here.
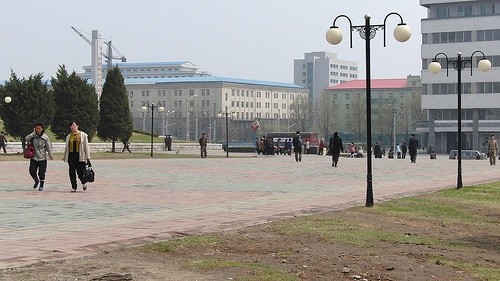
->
[264,131,320,147]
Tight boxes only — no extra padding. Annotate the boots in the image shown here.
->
[39,180,44,190]
[33,177,39,188]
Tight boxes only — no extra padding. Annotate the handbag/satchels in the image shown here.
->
[298,136,304,145]
[80,160,95,183]
[326,145,335,155]
[23,144,35,158]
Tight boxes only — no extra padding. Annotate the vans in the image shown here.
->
[449,149,480,160]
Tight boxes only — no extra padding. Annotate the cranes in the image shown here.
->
[71,25,127,74]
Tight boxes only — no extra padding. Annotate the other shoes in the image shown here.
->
[71,188,76,192]
[82,183,87,191]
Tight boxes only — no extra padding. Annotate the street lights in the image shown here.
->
[217,106,237,158]
[0,95,12,106]
[324,11,410,207]
[392,107,399,155]
[427,50,492,189]
[141,102,165,157]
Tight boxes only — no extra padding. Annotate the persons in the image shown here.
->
[396,144,401,159]
[63,120,90,192]
[25,123,54,190]
[293,131,303,162]
[122,140,130,152]
[330,132,344,167]
[388,149,393,158]
[375,142,382,158]
[408,134,419,163]
[305,140,309,154]
[476,154,480,160]
[430,151,436,159]
[402,143,407,159]
[358,147,363,155]
[199,133,208,159]
[318,140,324,155]
[165,135,172,151]
[350,142,356,157]
[488,135,499,165]
[0,131,8,153]
[255,137,292,155]
